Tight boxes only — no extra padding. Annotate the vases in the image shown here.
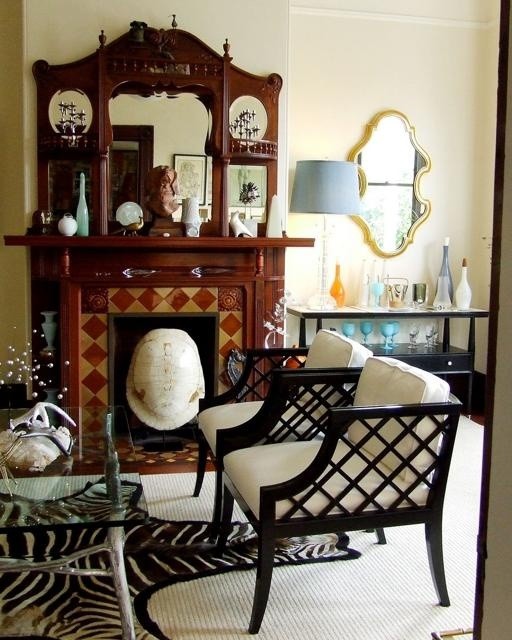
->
[0,403,79,477]
[433,274,453,308]
[436,246,455,302]
[453,266,472,311]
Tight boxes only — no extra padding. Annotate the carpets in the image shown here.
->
[2,414,487,640]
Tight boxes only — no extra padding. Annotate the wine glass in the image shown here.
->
[342,322,356,339]
[380,324,394,350]
[425,326,435,348]
[372,282,385,308]
[388,321,401,347]
[360,321,374,347]
[408,326,420,349]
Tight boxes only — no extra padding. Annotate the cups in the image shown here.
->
[412,283,427,310]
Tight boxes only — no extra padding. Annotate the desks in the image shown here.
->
[289,303,488,415]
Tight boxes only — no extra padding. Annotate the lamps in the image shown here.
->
[288,158,363,308]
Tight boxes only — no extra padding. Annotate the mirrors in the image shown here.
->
[46,160,91,222]
[229,164,267,224]
[48,87,92,140]
[342,109,433,257]
[103,79,219,234]
[227,94,270,146]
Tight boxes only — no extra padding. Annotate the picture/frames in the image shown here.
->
[172,154,209,208]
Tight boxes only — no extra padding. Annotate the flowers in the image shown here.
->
[1,324,75,431]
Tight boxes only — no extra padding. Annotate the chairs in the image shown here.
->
[215,354,467,640]
[195,326,378,544]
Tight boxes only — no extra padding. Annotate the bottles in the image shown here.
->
[358,259,369,306]
[435,237,454,305]
[455,258,472,310]
[379,259,388,306]
[369,259,379,306]
